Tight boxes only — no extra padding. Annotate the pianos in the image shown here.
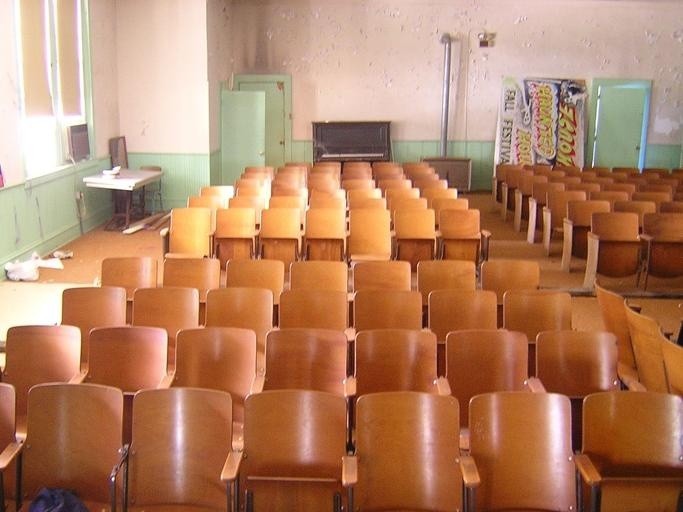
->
[312,121,391,162]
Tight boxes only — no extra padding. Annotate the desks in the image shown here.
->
[82,168,163,230]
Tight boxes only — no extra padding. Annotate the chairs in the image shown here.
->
[1,256,683,511]
[159,160,682,292]
